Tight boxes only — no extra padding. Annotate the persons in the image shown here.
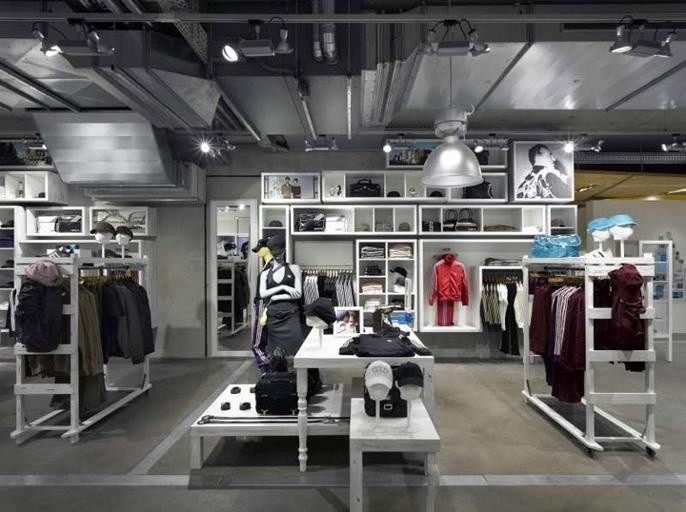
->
[608,262,646,350]
[281,177,292,198]
[517,145,572,198]
[291,178,302,198]
[252,235,307,374]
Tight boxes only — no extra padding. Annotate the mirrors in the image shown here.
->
[205,198,258,359]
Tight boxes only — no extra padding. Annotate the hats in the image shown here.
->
[224,243,236,251]
[390,266,407,277]
[398,362,423,386]
[302,297,336,325]
[552,219,565,227]
[26,259,63,288]
[251,235,285,254]
[587,214,636,232]
[365,360,394,389]
[90,220,133,242]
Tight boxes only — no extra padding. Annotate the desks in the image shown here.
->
[190,326,440,512]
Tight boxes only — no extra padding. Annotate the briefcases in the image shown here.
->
[255,371,297,415]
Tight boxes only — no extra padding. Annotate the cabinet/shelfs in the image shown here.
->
[521,255,662,457]
[0,165,156,446]
[217,259,250,337]
[258,204,578,332]
[260,141,577,202]
[616,239,674,361]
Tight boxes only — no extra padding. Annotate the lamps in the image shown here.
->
[29,16,119,57]
[419,19,491,57]
[419,1,484,189]
[609,15,677,59]
[220,16,292,62]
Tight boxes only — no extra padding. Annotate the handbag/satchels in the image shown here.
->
[531,234,582,257]
[443,208,479,231]
[349,178,381,197]
[462,180,492,198]
[364,387,407,417]
